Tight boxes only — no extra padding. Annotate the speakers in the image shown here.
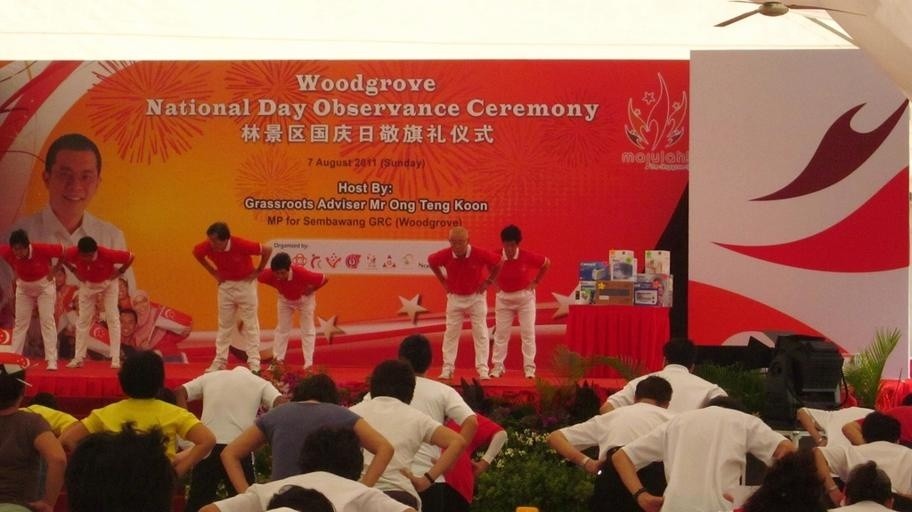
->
[692,346,773,372]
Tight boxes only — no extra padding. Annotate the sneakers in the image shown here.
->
[46,359,121,370]
[438,365,536,381]
[204,359,283,373]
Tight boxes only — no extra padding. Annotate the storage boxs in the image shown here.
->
[573,247,676,309]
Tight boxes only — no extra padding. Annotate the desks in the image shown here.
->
[566,304,671,379]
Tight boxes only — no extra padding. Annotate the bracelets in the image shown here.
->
[119,267,125,273]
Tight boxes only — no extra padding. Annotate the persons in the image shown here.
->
[191,220,273,375]
[257,251,329,372]
[0,132,191,361]
[61,234,135,369]
[0,336,509,512]
[1,230,64,369]
[548,334,912,512]
[489,224,551,380]
[430,226,503,381]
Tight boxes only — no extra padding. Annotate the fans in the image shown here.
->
[710,0,867,26]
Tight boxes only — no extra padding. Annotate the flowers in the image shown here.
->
[261,366,370,415]
[458,379,595,511]
